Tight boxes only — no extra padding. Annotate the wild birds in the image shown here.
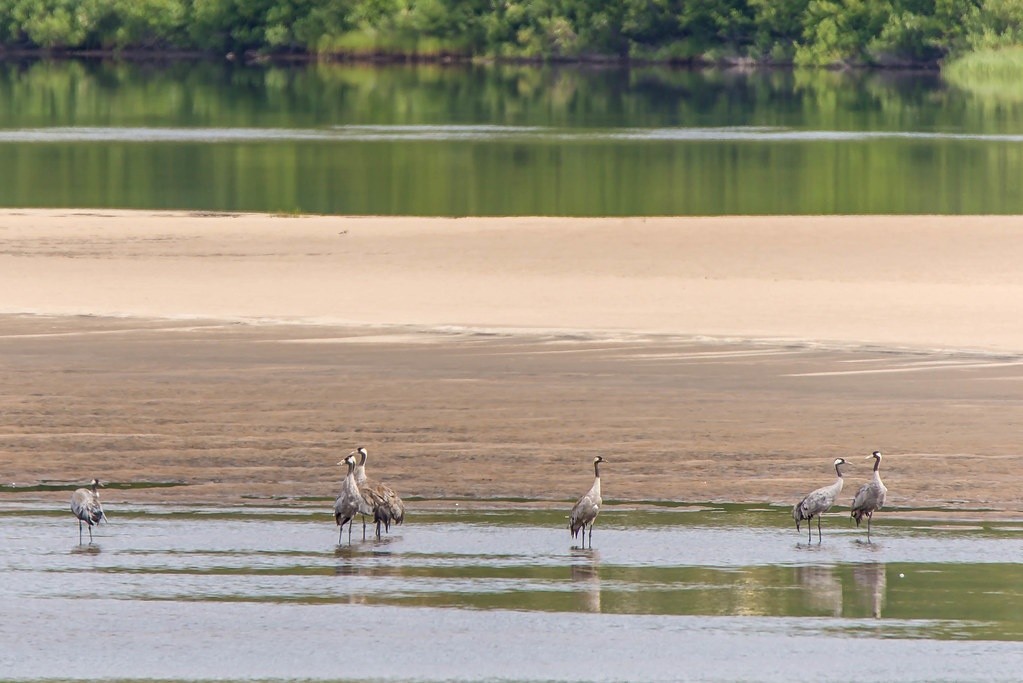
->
[567,456,609,549]
[334,454,393,546]
[350,447,405,541]
[791,457,852,546]
[850,451,888,542]
[70,477,110,544]
